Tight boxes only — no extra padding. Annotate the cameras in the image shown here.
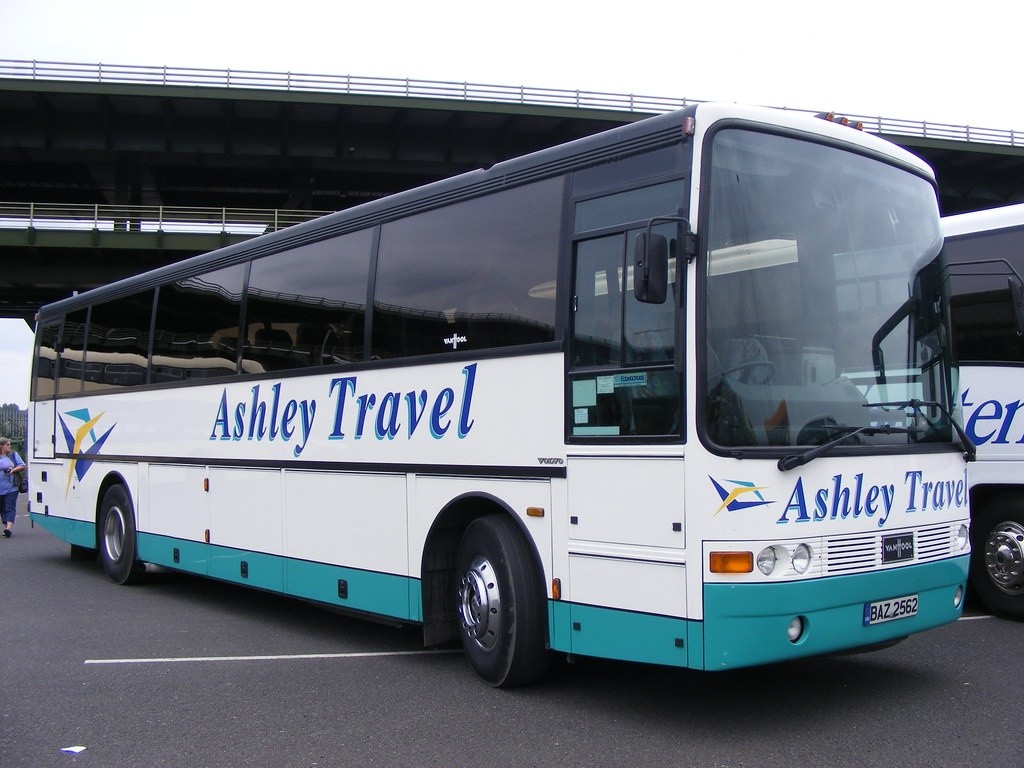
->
[4,468,11,473]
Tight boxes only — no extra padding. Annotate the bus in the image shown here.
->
[526,201,1024,622]
[25,99,976,693]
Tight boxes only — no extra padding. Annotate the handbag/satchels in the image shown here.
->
[12,450,27,494]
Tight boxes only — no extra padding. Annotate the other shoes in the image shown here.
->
[4,528,12,537]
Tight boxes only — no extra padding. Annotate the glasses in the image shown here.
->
[6,443,11,446]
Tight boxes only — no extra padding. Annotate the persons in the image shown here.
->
[0,437,26,537]
